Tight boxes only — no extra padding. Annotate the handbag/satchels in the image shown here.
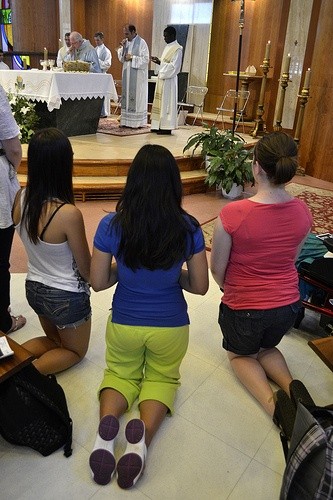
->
[0,364,73,457]
[277,400,333,499]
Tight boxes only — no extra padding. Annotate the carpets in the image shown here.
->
[199,183,333,253]
[1,268,333,500]
[96,118,156,137]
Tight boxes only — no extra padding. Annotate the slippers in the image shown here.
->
[273,379,315,440]
[8,314,26,334]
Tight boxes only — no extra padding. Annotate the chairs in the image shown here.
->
[212,90,251,132]
[109,79,123,115]
[176,86,209,128]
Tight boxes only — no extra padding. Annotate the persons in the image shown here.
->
[94,32,111,117]
[10,129,92,375]
[56,33,71,68]
[150,26,184,135]
[209,127,315,440]
[118,24,149,128]
[89,143,210,490]
[64,31,100,73]
[0,85,27,335]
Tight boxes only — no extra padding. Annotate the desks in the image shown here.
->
[223,72,266,122]
[0,69,119,138]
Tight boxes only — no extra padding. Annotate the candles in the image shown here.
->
[303,67,312,89]
[283,52,291,73]
[264,40,272,59]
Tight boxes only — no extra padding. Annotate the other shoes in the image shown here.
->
[89,414,148,490]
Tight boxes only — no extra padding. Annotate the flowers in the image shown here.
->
[6,93,41,144]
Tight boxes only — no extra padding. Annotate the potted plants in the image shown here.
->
[182,124,255,199]
